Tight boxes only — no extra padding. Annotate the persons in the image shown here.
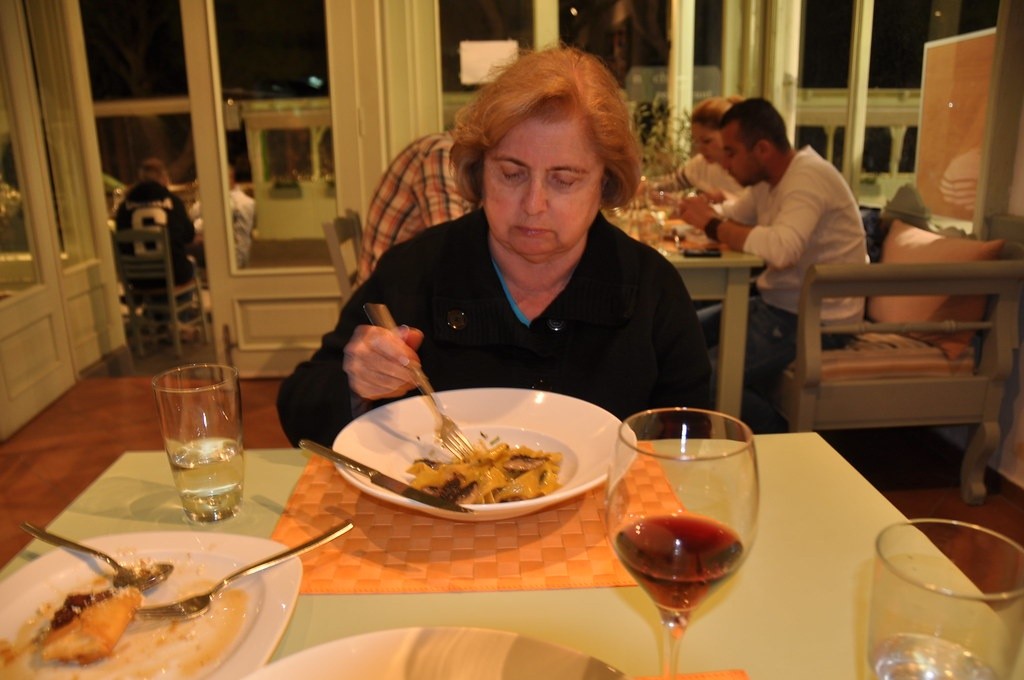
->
[111,158,195,323]
[679,97,871,410]
[651,95,745,204]
[193,164,255,268]
[357,131,472,286]
[276,47,716,451]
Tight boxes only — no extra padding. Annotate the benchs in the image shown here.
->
[774,212,1024,506]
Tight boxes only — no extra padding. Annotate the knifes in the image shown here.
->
[298,439,476,514]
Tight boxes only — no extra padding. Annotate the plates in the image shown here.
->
[241,627,625,680]
[0,529,304,680]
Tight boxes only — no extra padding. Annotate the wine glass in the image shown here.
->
[602,405,759,680]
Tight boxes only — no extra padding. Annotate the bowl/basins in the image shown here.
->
[331,388,637,523]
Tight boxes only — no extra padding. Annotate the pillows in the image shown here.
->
[863,217,1003,358]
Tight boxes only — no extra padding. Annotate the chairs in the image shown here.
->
[112,224,209,362]
[320,208,363,298]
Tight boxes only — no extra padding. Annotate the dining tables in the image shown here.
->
[1,433,1024,680]
[614,205,764,424]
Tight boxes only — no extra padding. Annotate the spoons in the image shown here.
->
[17,518,173,592]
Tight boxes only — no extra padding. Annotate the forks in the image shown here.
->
[376,305,475,460]
[135,520,353,622]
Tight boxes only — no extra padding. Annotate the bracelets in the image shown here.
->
[705,218,722,241]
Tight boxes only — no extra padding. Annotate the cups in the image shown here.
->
[864,519,1024,680]
[150,363,246,526]
[634,210,667,252]
